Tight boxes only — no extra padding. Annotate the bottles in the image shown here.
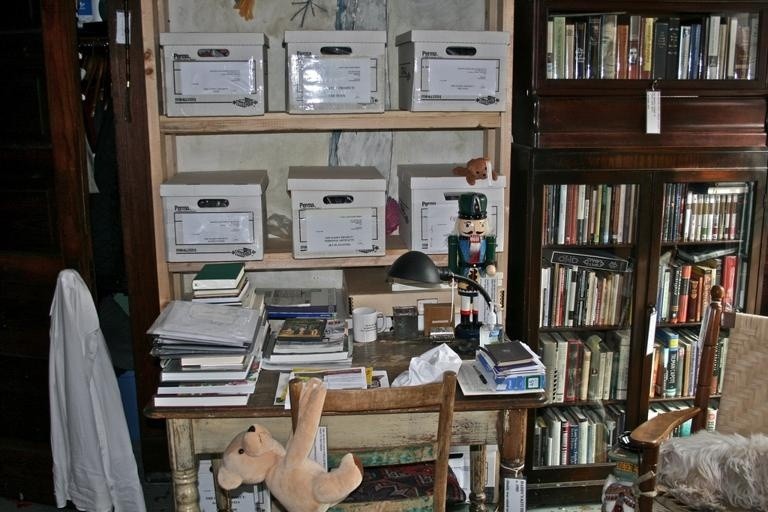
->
[454,296,483,354]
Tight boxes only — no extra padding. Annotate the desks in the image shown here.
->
[141,335,549,512]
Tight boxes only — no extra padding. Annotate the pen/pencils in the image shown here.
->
[473,365,487,384]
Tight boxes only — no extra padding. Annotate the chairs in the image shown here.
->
[289,370,456,512]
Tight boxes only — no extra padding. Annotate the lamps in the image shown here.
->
[384,250,503,349]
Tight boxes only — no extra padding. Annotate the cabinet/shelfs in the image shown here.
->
[508,0,768,507]
[140,0,516,336]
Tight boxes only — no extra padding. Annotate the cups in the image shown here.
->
[352,307,387,343]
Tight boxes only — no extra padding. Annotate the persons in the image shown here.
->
[444,190,496,337]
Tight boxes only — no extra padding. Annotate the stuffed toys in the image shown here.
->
[217,378,364,511]
[451,157,499,186]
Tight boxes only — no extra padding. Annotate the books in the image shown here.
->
[479,339,545,377]
[658,244,749,325]
[539,331,626,399]
[648,401,718,438]
[149,257,390,406]
[532,405,624,466]
[650,327,729,397]
[539,249,632,326]
[542,185,636,246]
[660,183,749,243]
[543,6,763,84]
